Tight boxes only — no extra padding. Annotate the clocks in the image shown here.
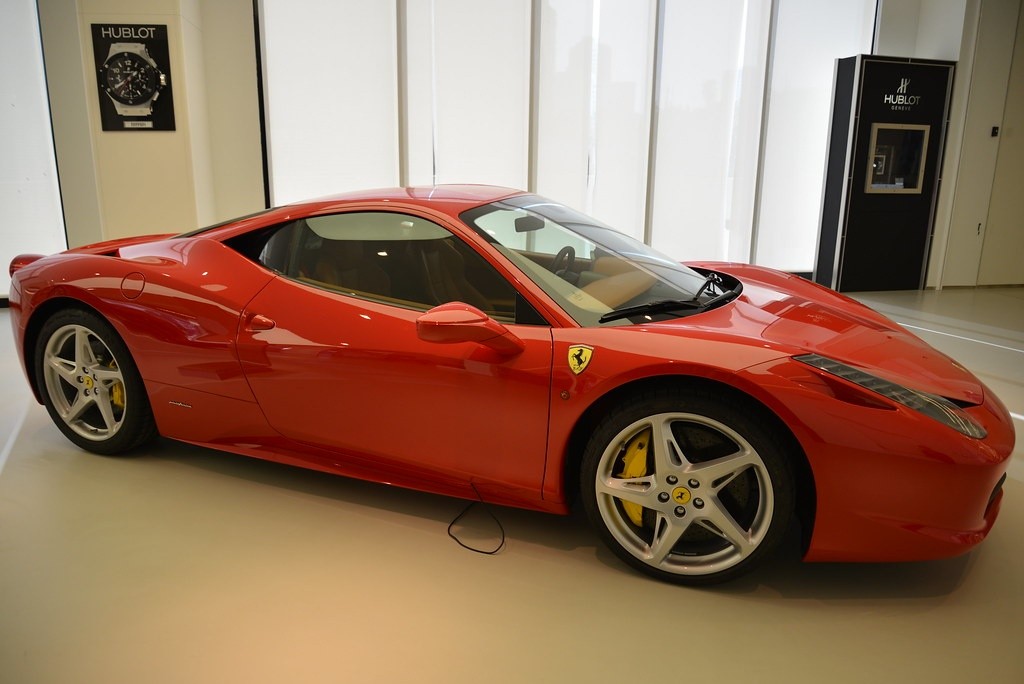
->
[103,52,157,106]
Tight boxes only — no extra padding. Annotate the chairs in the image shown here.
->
[311,238,391,295]
[416,238,495,311]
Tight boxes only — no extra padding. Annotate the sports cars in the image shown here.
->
[7,183,1018,587]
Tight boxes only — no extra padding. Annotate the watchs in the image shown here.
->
[100,43,167,117]
